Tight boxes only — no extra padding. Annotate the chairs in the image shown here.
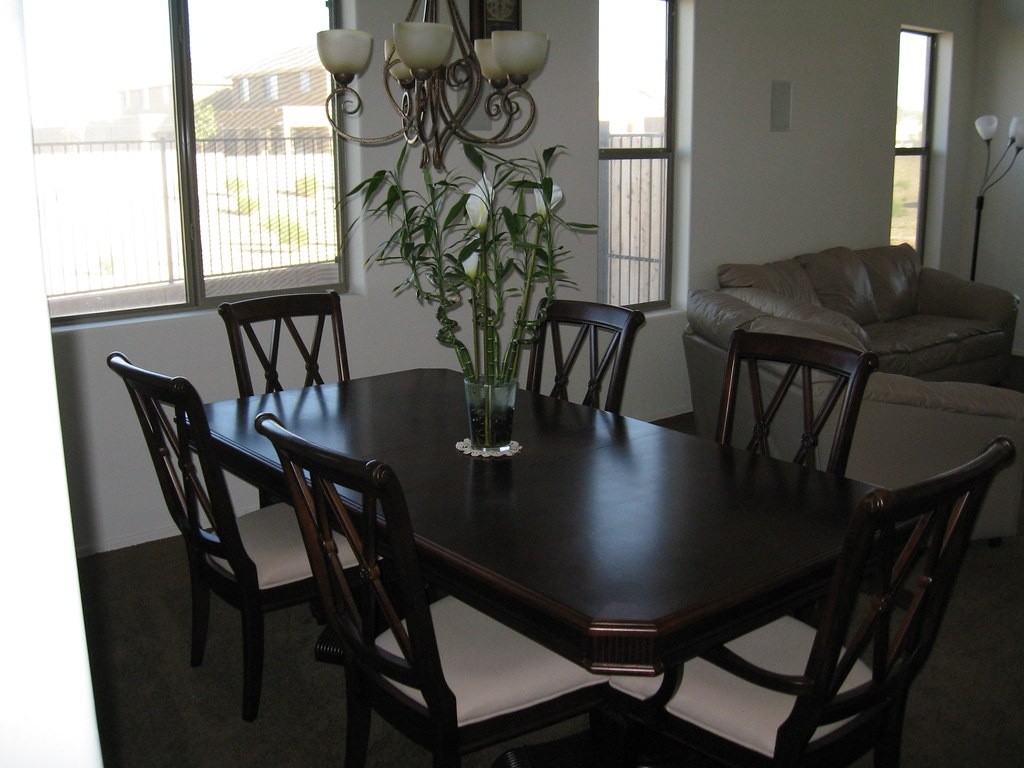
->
[255,412,610,768]
[109,351,389,723]
[526,297,646,419]
[217,291,352,398]
[718,329,881,478]
[592,437,1015,768]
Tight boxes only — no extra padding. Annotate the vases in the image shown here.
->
[463,375,520,452]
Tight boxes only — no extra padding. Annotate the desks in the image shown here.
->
[172,368,930,768]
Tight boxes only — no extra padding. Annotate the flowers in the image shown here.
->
[332,118,601,448]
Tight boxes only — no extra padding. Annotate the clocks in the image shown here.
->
[470,0,523,44]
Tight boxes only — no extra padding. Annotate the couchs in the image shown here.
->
[684,243,1024,545]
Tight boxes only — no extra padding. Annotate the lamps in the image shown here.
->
[317,0,549,169]
[970,116,1024,284]
